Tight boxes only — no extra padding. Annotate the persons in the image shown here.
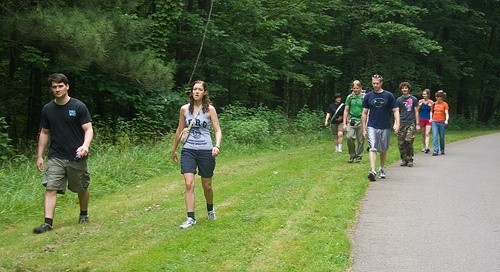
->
[418,89,433,153]
[172,81,222,228]
[324,94,348,152]
[34,74,93,233]
[362,75,400,181]
[343,80,365,163]
[393,82,420,166]
[431,92,449,155]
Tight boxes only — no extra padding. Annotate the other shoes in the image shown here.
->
[433,151,438,156]
[337,144,342,153]
[348,157,354,163]
[400,160,407,166]
[424,148,430,153]
[441,150,445,155]
[421,148,424,152]
[408,160,414,166]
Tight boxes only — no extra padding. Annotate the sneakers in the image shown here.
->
[206,207,217,220]
[179,217,196,229]
[79,215,89,223]
[369,170,376,182]
[33,223,53,233]
[379,167,386,179]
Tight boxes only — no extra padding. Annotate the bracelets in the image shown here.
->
[215,146,220,150]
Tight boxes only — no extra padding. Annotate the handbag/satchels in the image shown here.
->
[178,128,190,144]
